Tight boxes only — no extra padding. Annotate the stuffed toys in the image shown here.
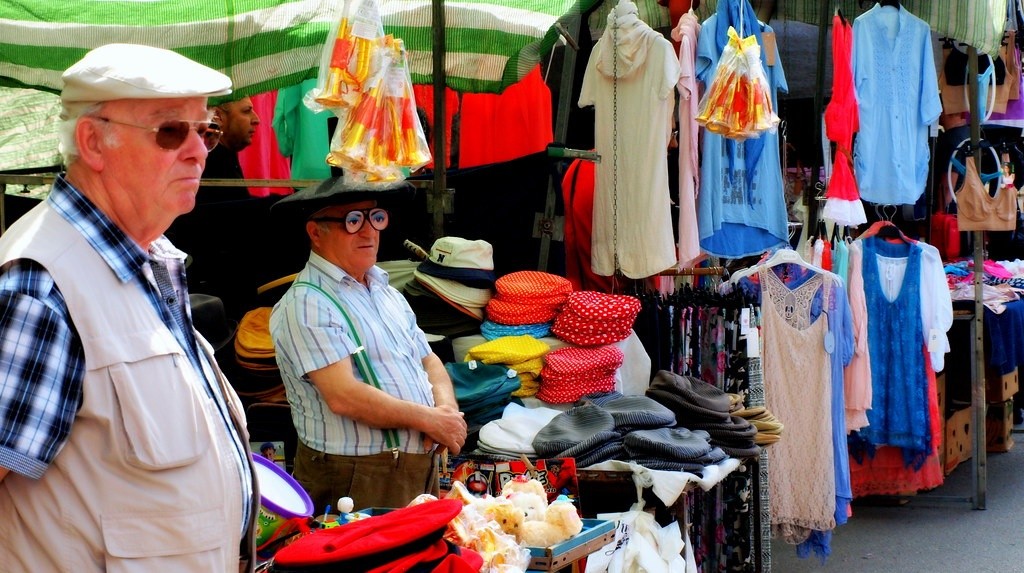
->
[466,475,583,549]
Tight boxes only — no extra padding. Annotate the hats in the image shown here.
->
[60,42,233,101]
[270,176,416,211]
[188,236,783,479]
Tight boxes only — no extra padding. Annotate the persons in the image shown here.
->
[270,199,468,516]
[0,44,262,573]
[195,95,260,205]
[562,105,678,295]
[937,94,983,256]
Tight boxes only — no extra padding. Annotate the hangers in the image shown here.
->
[623,178,918,312]
[936,29,1019,53]
[834,0,845,25]
[876,0,900,11]
[957,129,1024,159]
[687,0,697,19]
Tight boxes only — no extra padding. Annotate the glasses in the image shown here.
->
[311,207,389,233]
[87,115,223,152]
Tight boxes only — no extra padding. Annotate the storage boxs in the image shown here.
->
[954,403,972,464]
[943,411,959,475]
[935,374,946,481]
[986,366,1021,451]
